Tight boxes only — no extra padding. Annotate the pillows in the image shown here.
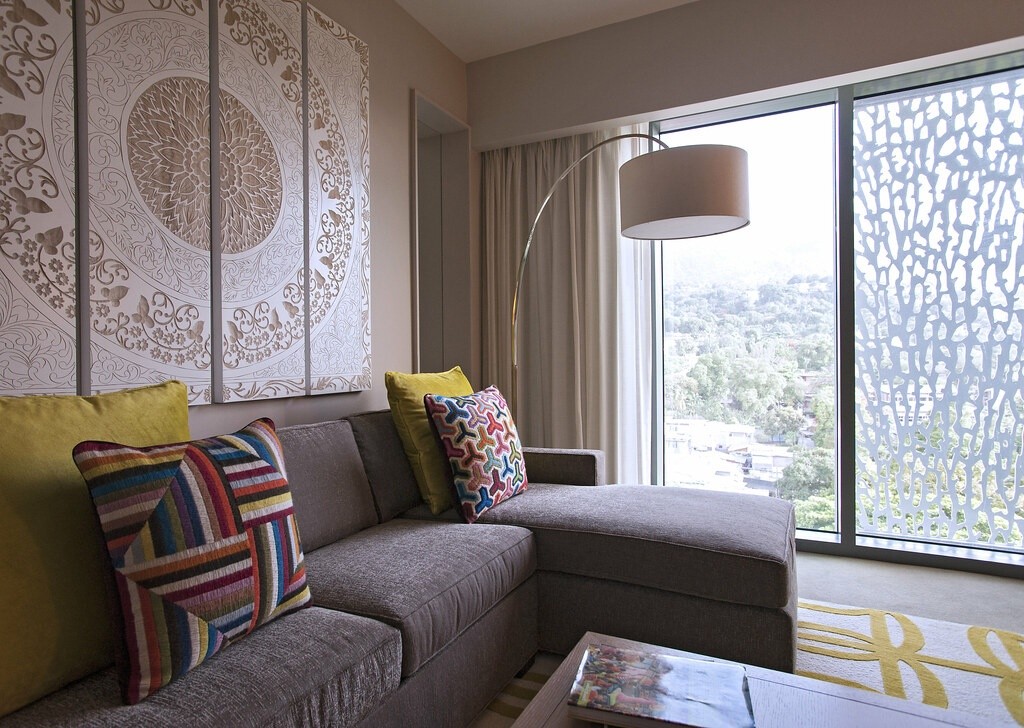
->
[425,384,528,523]
[72,417,315,704]
[385,366,476,519]
[0,379,190,719]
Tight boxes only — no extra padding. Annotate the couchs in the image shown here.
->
[0,410,799,728]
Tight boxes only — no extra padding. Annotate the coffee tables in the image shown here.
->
[510,630,986,728]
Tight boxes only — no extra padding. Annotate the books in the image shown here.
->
[566,644,757,728]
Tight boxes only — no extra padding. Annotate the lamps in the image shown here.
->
[512,133,750,433]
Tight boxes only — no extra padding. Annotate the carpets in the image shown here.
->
[480,596,1024,728]
